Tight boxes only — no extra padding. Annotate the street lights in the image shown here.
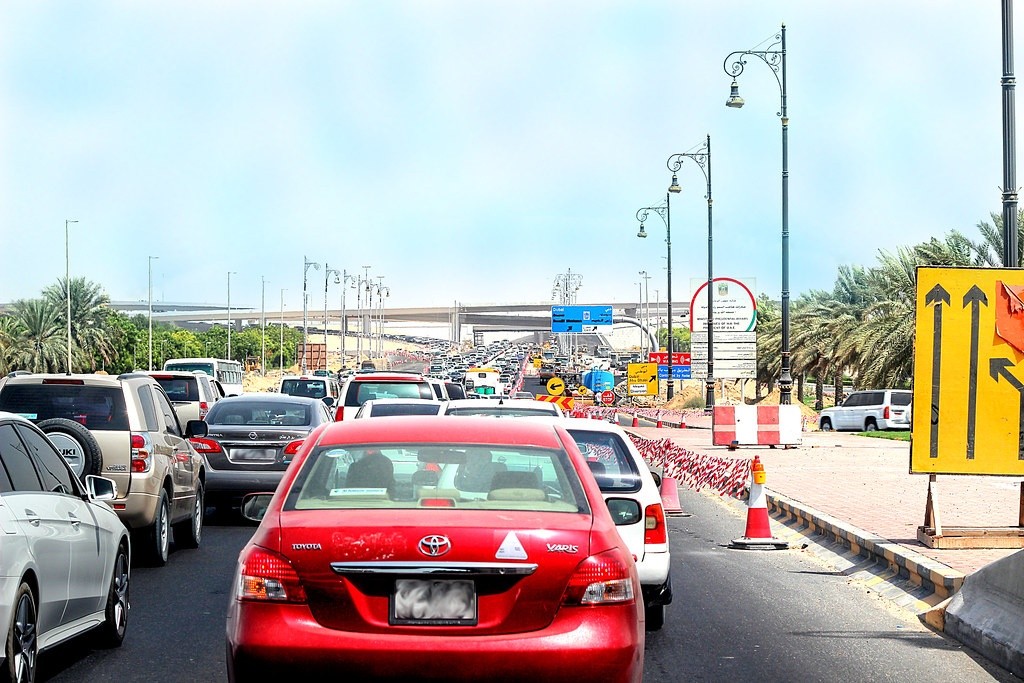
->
[665,134,716,415]
[227,272,288,378]
[634,191,674,401]
[302,256,391,375]
[148,256,162,371]
[723,22,793,404]
[66,219,78,371]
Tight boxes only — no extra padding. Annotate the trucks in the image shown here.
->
[578,368,614,393]
[465,368,500,396]
[297,343,327,373]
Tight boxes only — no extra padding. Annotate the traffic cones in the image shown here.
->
[729,456,790,549]
[574,409,687,428]
[657,438,693,518]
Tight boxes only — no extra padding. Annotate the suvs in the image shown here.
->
[332,370,451,422]
[0,368,225,566]
[817,389,912,432]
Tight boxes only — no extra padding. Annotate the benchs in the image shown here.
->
[335,488,544,501]
[225,414,301,425]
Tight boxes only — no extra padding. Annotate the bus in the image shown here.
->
[164,356,243,397]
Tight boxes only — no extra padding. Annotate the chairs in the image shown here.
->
[345,462,393,499]
[487,471,546,500]
[70,397,110,417]
[587,461,613,485]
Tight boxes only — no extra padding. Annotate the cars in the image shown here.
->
[371,331,638,394]
[195,376,674,683]
[0,411,132,683]
